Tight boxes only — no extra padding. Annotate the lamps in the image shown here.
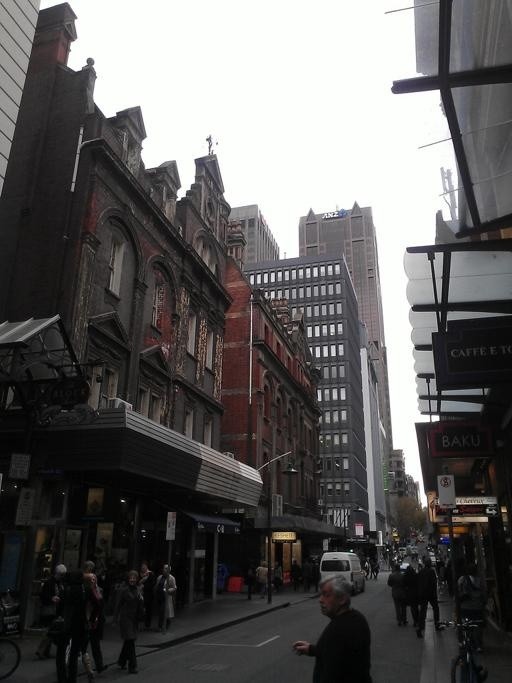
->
[257,450,300,476]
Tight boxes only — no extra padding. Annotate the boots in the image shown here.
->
[82,652,96,683]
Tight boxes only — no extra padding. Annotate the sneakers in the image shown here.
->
[117,659,139,674]
[97,663,108,674]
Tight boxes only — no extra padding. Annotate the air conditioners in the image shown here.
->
[107,397,133,412]
[222,451,235,459]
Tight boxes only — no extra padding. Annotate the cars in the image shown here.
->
[393,536,440,574]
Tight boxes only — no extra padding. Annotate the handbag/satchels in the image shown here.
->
[458,573,474,603]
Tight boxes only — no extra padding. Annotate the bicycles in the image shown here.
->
[433,615,490,682]
[0,588,23,682]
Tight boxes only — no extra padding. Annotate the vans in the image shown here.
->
[318,549,368,598]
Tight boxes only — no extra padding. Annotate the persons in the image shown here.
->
[293,574,369,682]
[364,557,380,581]
[246,556,317,599]
[386,551,445,637]
[35,556,175,682]
